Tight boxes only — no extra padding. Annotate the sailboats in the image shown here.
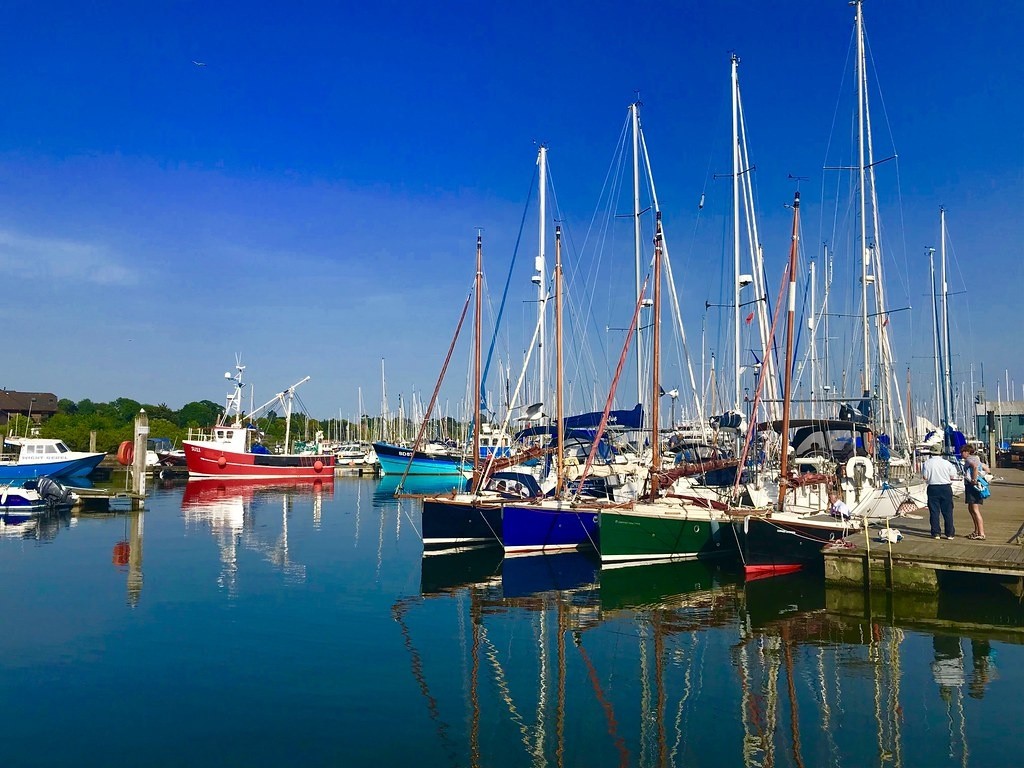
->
[145,0,1024,596]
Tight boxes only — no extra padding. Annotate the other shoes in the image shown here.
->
[969,532,977,538]
[932,533,941,541]
[946,535,956,541]
[974,535,988,540]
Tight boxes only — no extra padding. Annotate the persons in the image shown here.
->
[959,444,990,540]
[922,444,955,540]
[829,495,850,521]
[666,434,683,451]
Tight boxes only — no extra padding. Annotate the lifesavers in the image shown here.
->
[113,543,130,565]
[118,440,135,465]
[846,455,874,480]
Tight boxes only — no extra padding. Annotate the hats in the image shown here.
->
[931,444,942,454]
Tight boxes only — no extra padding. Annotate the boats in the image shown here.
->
[0,475,126,524]
[2,397,110,485]
